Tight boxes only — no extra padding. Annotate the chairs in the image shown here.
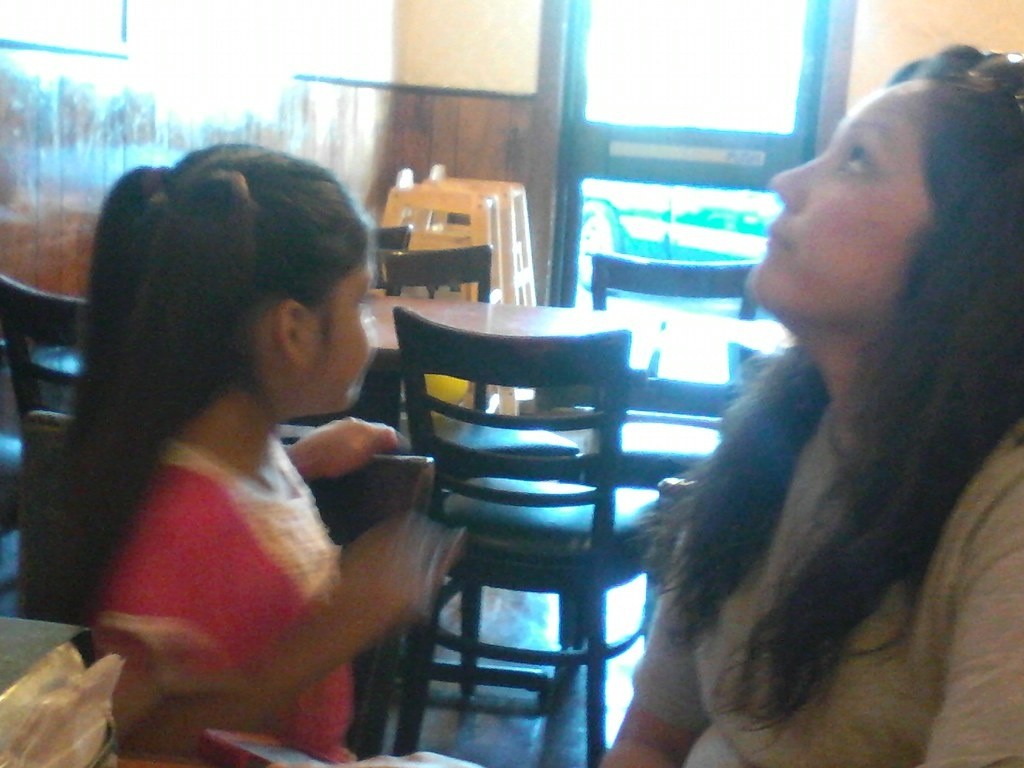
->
[0,167,767,768]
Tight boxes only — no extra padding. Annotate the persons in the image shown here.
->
[50,144,489,767]
[596,70,1024,767]
